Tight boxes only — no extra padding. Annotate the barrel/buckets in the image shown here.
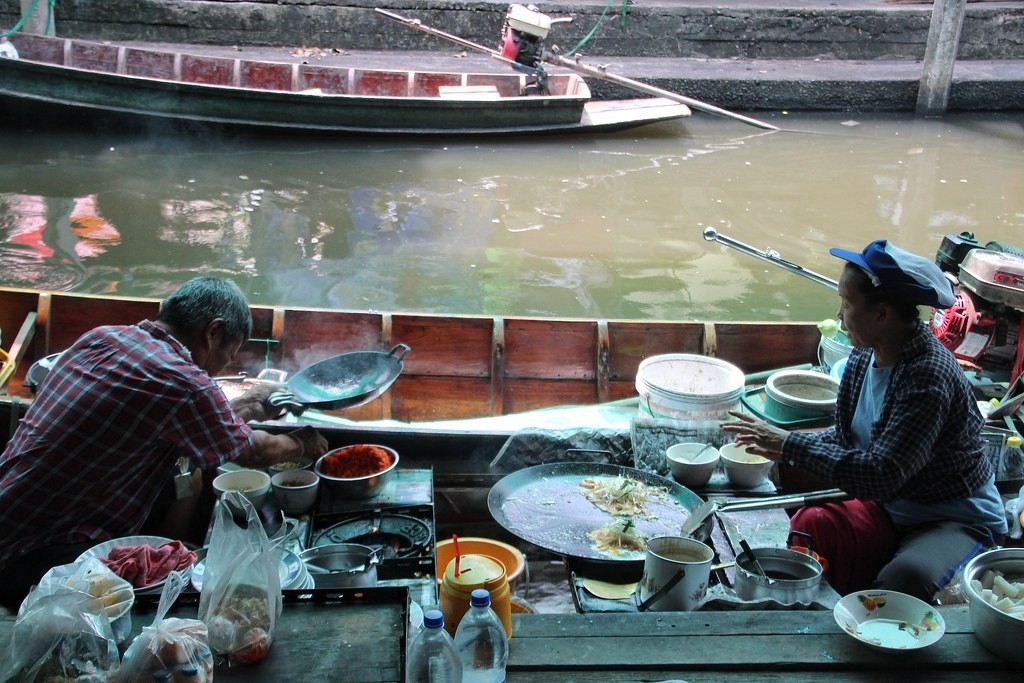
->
[816,333,853,373]
[430,537,530,600]
[21,353,61,394]
[740,369,839,432]
[635,352,746,422]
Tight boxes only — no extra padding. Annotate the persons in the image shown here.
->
[0,277,327,620]
[718,240,1009,607]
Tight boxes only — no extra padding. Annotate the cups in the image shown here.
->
[635,537,714,613]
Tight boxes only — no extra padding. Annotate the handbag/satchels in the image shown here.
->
[195,491,301,664]
[118,571,214,683]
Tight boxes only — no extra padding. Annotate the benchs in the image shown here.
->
[698,473,838,606]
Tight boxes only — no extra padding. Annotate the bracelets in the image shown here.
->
[287,433,305,458]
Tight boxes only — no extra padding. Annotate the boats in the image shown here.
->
[0,230,1022,433]
[0,0,690,135]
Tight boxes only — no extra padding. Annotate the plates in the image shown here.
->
[72,535,195,594]
[19,574,135,629]
[190,546,315,591]
[834,589,946,653]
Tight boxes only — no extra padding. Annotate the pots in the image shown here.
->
[294,544,386,587]
[487,449,714,564]
[266,343,412,412]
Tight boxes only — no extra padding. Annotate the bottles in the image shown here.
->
[451,588,509,682]
[1000,437,1024,480]
[439,554,513,659]
[405,610,462,683]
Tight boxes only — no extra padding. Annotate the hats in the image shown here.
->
[828,240,956,309]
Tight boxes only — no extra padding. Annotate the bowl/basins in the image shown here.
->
[313,443,400,501]
[270,458,314,474]
[734,549,823,604]
[212,469,271,517]
[719,442,775,489]
[666,443,720,489]
[271,469,321,515]
[963,548,1024,664]
